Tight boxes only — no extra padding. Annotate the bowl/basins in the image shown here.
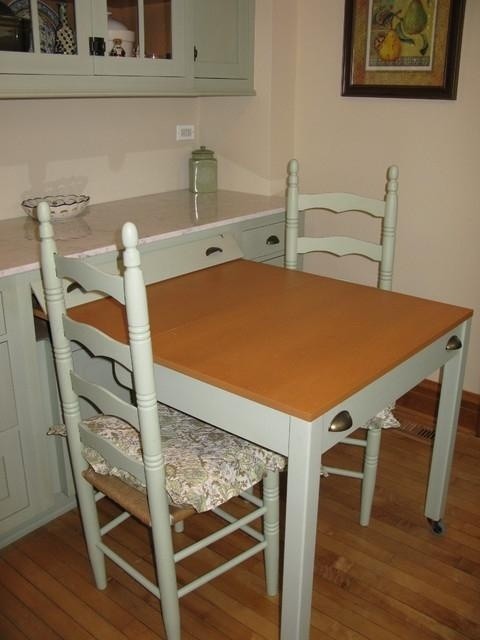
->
[20,193,91,223]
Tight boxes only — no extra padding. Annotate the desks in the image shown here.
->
[61,257,474,639]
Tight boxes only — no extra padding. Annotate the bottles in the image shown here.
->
[188,143,218,193]
[54,0,75,55]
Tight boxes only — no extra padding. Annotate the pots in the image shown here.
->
[106,11,136,56]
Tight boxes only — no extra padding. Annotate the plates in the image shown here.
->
[8,0,63,53]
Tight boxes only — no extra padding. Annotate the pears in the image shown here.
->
[403,0,426,34]
[379,30,401,60]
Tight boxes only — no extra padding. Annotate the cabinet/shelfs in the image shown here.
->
[1,0,253,96]
[2,208,305,548]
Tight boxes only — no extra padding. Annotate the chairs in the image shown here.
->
[39,201,290,637]
[243,163,397,528]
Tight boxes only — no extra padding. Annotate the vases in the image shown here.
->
[341,0,465,101]
